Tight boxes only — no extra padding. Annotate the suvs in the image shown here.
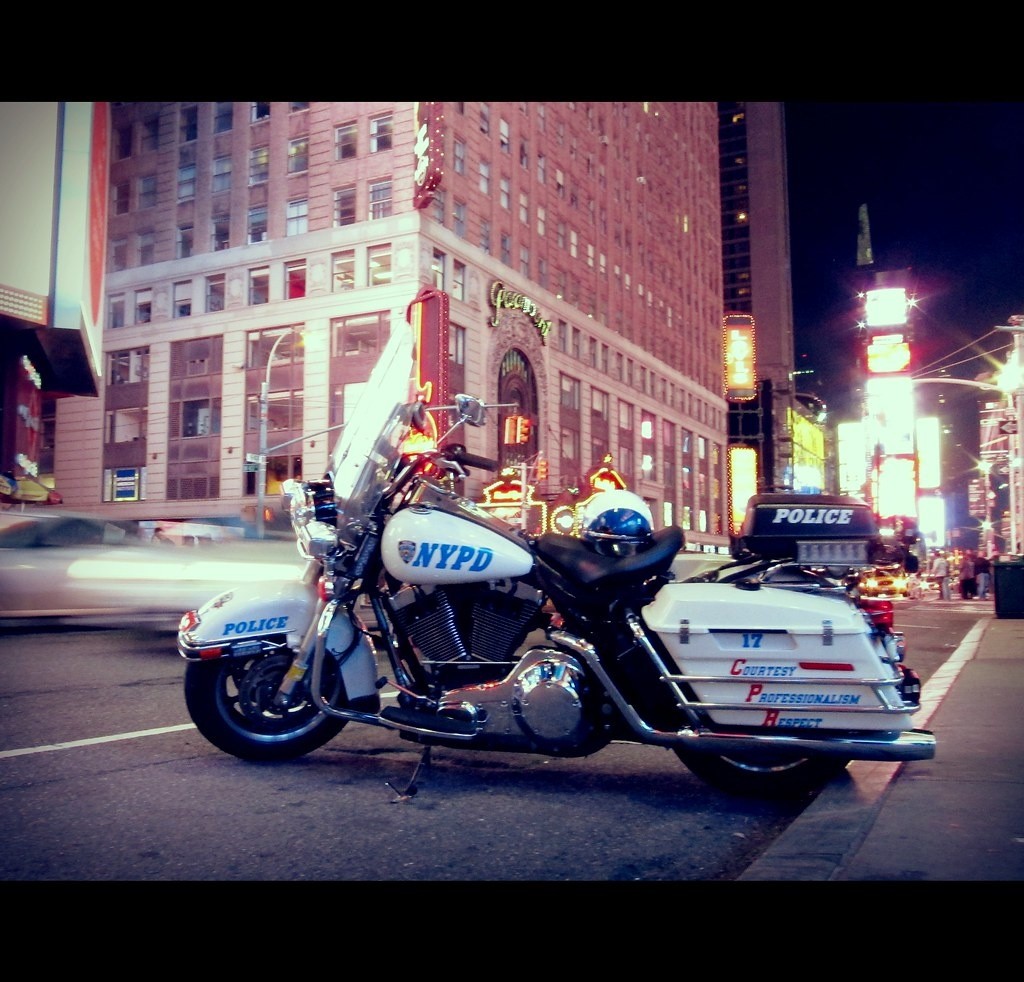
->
[866,567,911,596]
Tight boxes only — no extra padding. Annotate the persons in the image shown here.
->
[930,548,992,601]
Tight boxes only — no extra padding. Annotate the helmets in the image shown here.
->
[583,490,657,557]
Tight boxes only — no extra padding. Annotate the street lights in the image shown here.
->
[789,392,827,493]
[255,326,309,540]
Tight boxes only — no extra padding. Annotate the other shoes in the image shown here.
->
[936,597,952,602]
[961,593,992,600]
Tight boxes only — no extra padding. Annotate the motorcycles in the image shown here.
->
[177,396,940,804]
